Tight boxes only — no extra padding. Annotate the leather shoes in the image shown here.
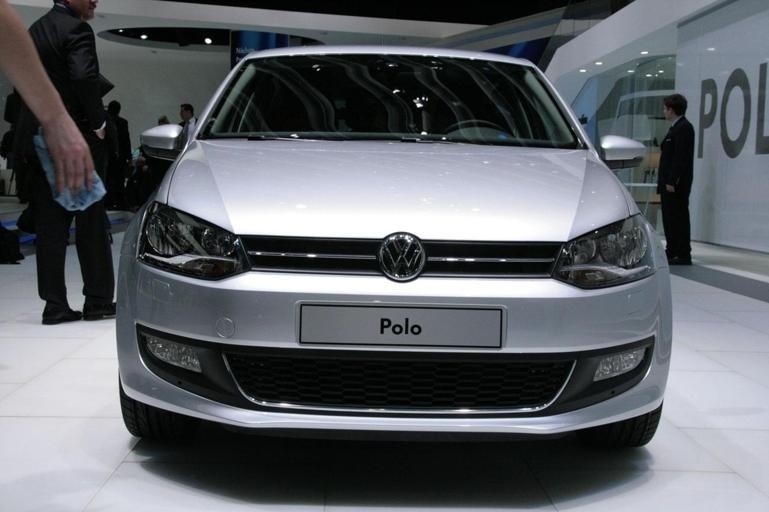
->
[41,305,82,325]
[81,301,116,322]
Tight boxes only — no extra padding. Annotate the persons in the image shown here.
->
[657,94,695,265]
[177,103,199,142]
[0,126,30,203]
[106,100,131,209]
[22,2,119,324]
[1,1,97,198]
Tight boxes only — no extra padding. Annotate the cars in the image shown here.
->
[606,90,671,178]
[112,44,672,453]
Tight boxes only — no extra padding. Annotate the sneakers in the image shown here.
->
[668,256,692,265]
